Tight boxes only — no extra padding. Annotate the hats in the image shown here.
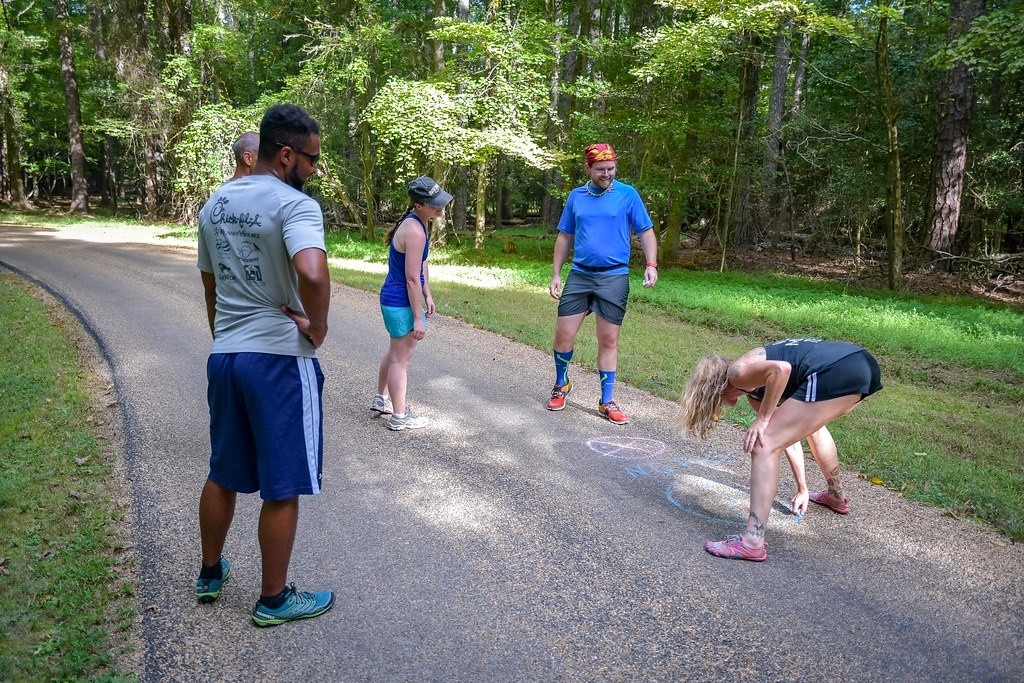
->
[408,176,453,208]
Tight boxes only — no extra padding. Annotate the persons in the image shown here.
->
[372,178,453,430]
[197,105,337,628]
[548,141,658,422]
[679,337,883,562]
[219,131,260,181]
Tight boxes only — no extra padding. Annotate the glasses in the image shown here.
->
[277,142,322,168]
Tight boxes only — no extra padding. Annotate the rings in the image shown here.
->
[646,262,658,268]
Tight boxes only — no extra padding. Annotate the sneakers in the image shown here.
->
[195,558,230,603]
[388,404,429,431]
[809,490,849,514]
[547,379,573,410]
[252,586,337,628]
[598,398,629,425]
[370,393,393,414]
[705,534,769,563]
